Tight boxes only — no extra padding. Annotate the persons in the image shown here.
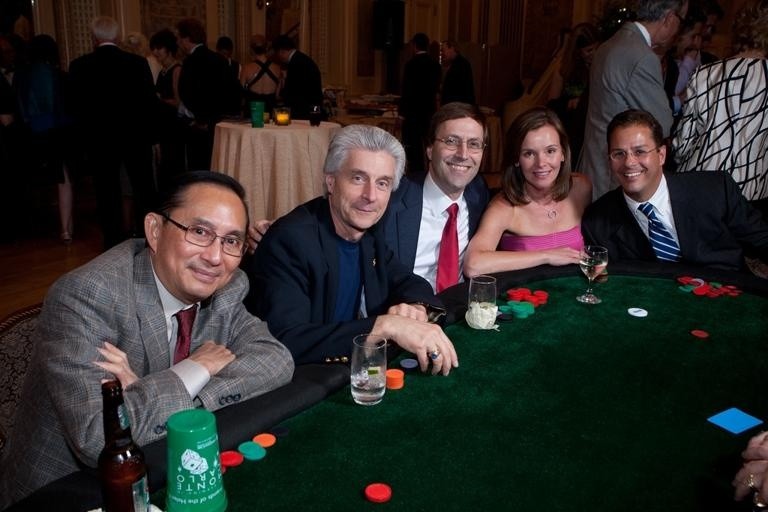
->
[387,31,477,191]
[516,0,767,200]
[237,124,460,378]
[2,171,296,511]
[731,427,766,510]
[581,107,766,275]
[460,107,594,279]
[0,15,321,243]
[243,101,495,298]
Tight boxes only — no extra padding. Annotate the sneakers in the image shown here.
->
[56,232,72,240]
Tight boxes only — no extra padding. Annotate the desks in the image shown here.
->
[210,118,343,228]
[1,263,768,510]
[337,92,503,191]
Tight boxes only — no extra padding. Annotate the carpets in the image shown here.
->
[0,300,44,509]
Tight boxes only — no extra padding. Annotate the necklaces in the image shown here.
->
[525,193,564,222]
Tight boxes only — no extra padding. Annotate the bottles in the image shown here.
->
[97,380,151,511]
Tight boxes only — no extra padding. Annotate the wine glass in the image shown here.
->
[576,245,609,304]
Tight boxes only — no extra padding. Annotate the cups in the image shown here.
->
[164,409,229,511]
[350,334,387,405]
[468,276,497,330]
[275,108,291,125]
[309,106,321,125]
[250,102,264,128]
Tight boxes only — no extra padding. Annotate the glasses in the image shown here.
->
[673,11,684,24]
[166,216,249,257]
[434,136,488,153]
[609,147,658,160]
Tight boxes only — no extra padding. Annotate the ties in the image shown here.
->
[638,202,681,264]
[435,204,459,295]
[174,304,197,364]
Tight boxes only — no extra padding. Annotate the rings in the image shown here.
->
[745,473,754,488]
[750,492,765,509]
[428,350,440,360]
